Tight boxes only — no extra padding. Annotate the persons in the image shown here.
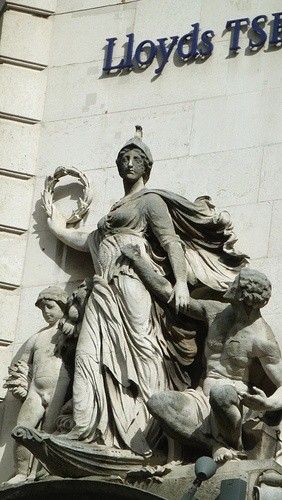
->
[4,134,281,491]
